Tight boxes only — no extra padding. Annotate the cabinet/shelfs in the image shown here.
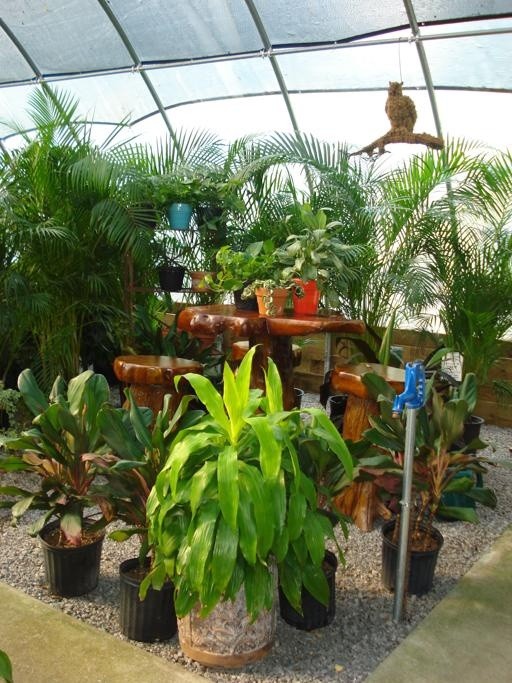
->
[120,207,228,411]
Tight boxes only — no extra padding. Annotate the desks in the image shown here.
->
[173,302,366,415]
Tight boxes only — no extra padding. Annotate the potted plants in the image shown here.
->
[0,337,501,671]
[149,168,368,319]
